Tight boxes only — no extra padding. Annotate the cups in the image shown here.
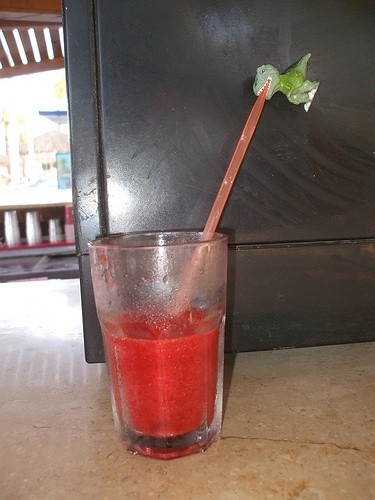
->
[26,211,42,246]
[3,210,21,248]
[88,228,228,460]
[65,204,75,243]
[49,218,62,245]
